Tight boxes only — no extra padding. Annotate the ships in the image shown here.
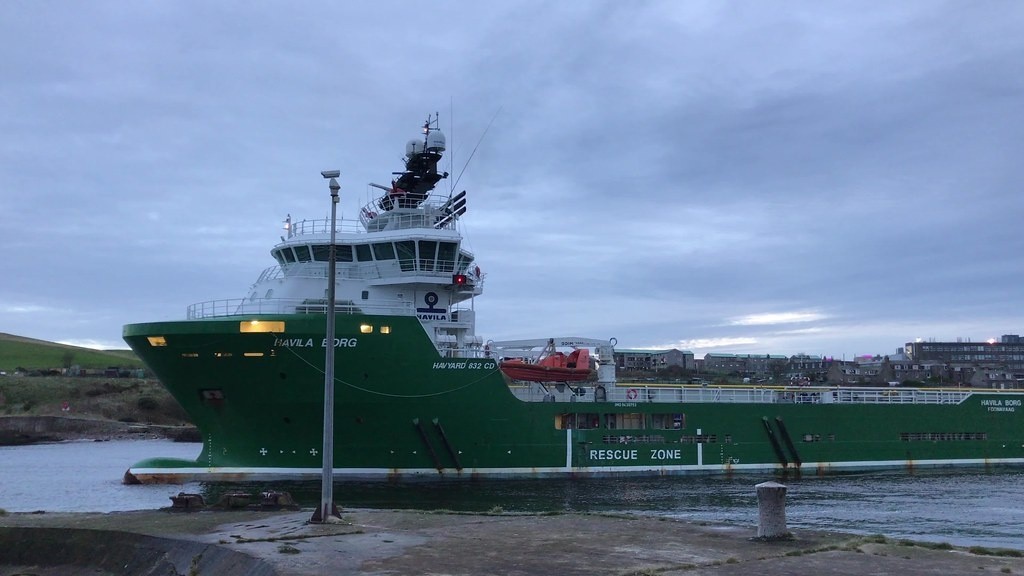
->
[119,106,1024,490]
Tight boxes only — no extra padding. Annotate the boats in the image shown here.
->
[492,336,594,381]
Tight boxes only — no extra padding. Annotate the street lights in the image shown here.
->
[318,168,349,524]
